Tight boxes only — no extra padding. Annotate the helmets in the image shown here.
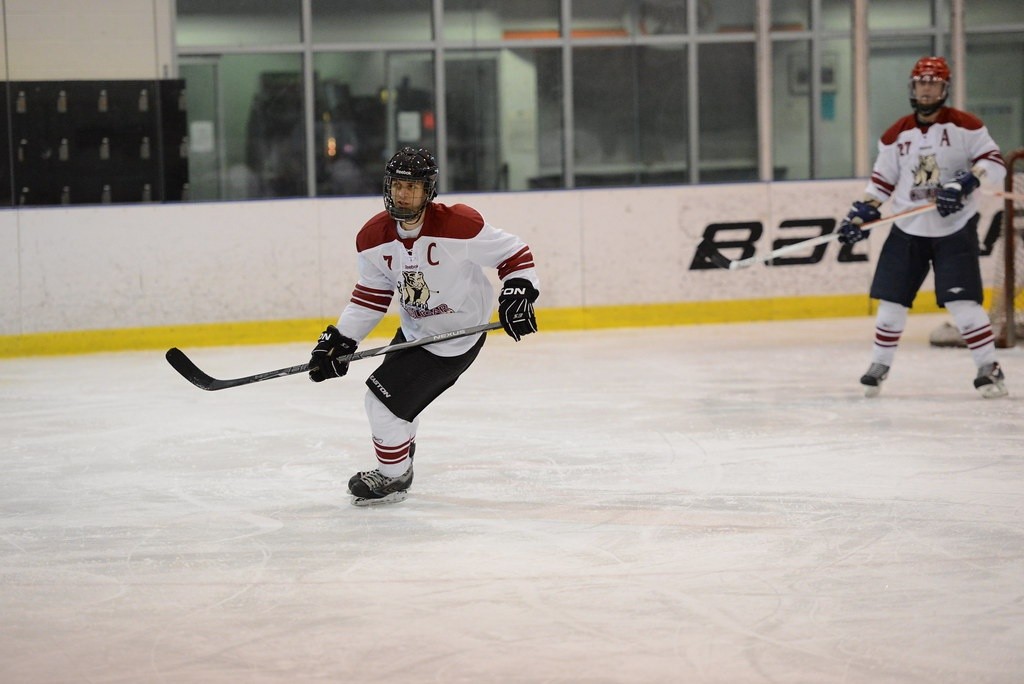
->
[910,57,952,117]
[383,146,437,221]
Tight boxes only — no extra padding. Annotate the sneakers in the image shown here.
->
[347,467,383,494]
[860,362,890,397]
[352,459,413,506]
[974,362,1008,398]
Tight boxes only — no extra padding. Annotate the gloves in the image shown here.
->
[308,324,358,383]
[837,201,881,243]
[935,170,980,217]
[498,278,540,341]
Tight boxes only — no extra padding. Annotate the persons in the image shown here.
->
[309,147,539,506]
[837,58,1010,399]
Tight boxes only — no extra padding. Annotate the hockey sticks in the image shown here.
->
[727,187,948,271]
[166,321,505,392]
[982,188,1024,199]
